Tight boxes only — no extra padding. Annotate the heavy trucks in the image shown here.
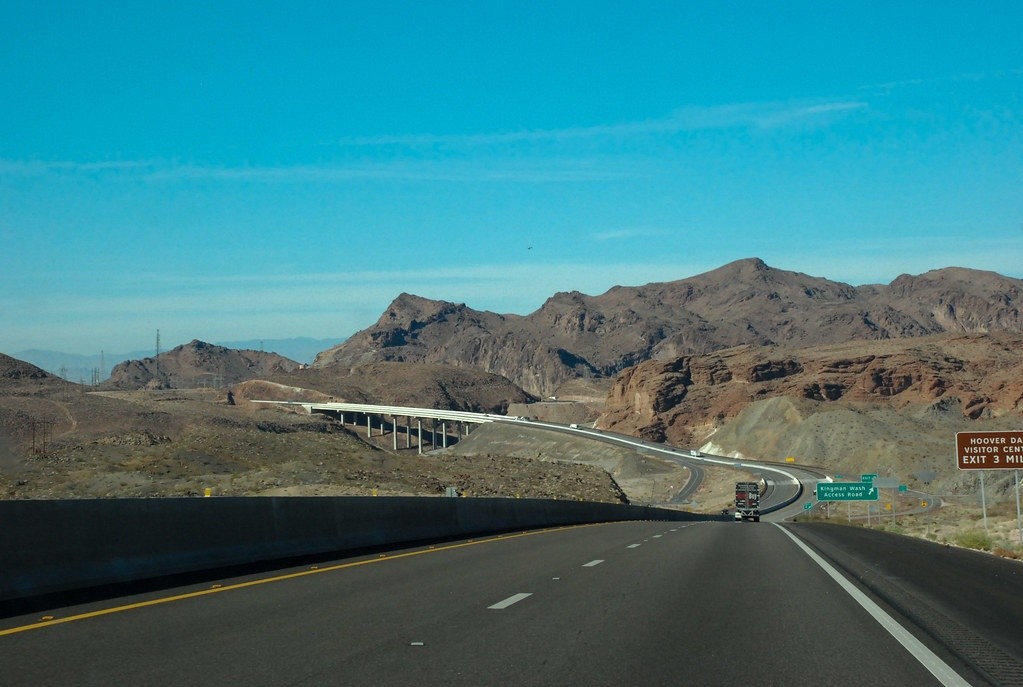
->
[735,482,760,522]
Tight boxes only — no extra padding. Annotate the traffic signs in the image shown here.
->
[816,482,879,501]
